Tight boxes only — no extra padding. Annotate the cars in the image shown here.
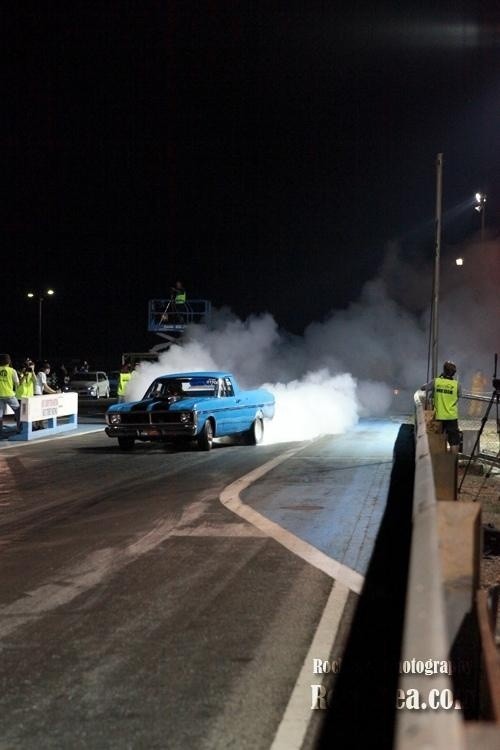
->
[105,370,119,396]
[65,371,110,399]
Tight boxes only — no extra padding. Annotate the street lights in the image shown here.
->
[470,191,490,240]
[26,289,56,361]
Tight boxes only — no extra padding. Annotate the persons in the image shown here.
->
[79,360,90,373]
[170,281,189,324]
[116,362,132,404]
[467,367,488,418]
[420,360,463,458]
[0,353,70,435]
[121,362,132,372]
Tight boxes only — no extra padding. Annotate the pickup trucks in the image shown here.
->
[102,370,278,453]
